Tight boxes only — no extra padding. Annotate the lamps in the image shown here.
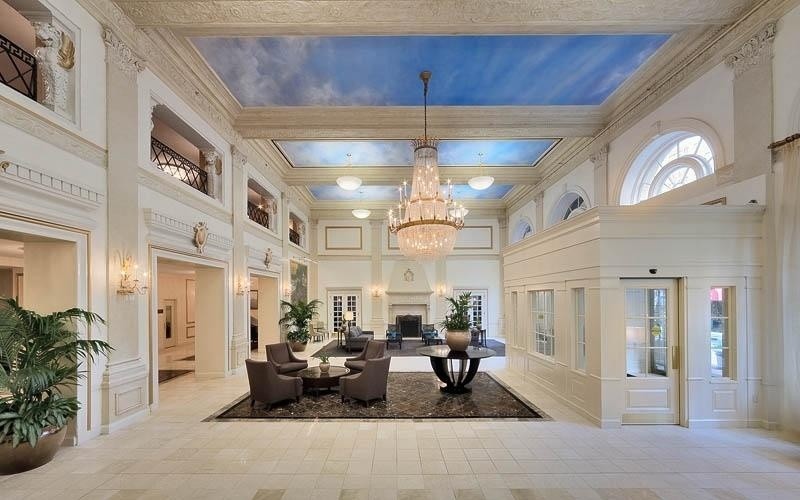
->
[369,286,383,298]
[343,310,354,330]
[117,254,150,296]
[384,69,467,262]
[334,152,363,192]
[283,285,292,296]
[468,151,495,192]
[430,284,453,297]
[350,187,372,218]
[236,277,250,294]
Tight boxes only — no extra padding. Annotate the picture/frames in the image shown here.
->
[249,289,258,310]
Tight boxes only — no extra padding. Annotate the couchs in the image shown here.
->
[343,324,375,354]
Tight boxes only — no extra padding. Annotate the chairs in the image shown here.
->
[266,341,309,379]
[308,321,323,344]
[420,323,439,342]
[385,323,402,338]
[344,339,387,372]
[315,316,331,339]
[244,356,304,413]
[337,353,390,406]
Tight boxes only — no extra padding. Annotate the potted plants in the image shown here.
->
[317,352,331,372]
[1,290,117,476]
[278,298,324,353]
[441,286,478,352]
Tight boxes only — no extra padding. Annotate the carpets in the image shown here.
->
[309,337,510,360]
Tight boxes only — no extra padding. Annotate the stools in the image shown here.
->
[385,338,402,350]
[424,337,445,345]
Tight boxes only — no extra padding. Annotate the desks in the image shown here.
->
[292,365,353,402]
[478,329,487,349]
[413,343,497,395]
[336,326,346,349]
